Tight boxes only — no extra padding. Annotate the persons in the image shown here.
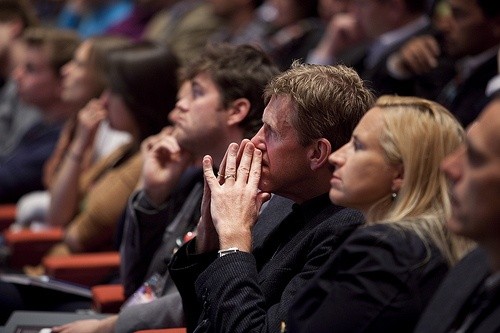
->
[0,0,500,333]
